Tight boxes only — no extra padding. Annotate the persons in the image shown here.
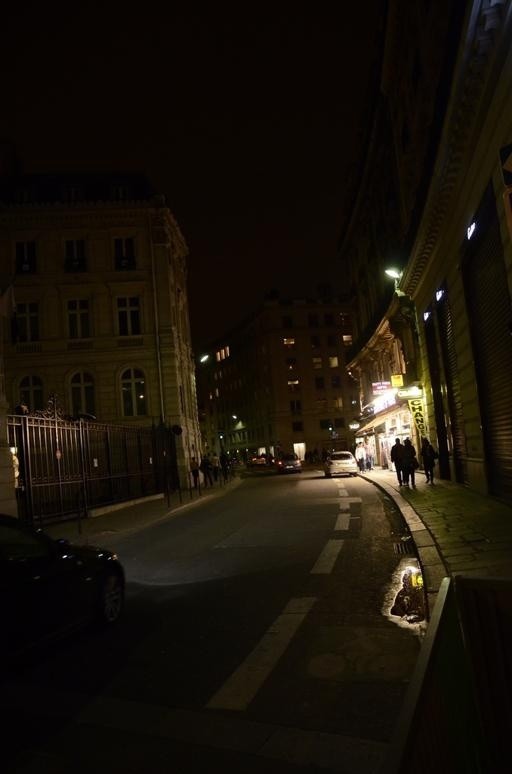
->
[390,438,438,488]
[190,451,231,488]
[355,439,375,474]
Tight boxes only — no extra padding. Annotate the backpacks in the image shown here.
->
[432,452,439,460]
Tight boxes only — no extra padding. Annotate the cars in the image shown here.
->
[324,451,358,478]
[0,512,126,654]
[279,453,302,473]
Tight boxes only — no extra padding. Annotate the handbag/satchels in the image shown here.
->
[408,457,419,471]
[366,462,371,468]
